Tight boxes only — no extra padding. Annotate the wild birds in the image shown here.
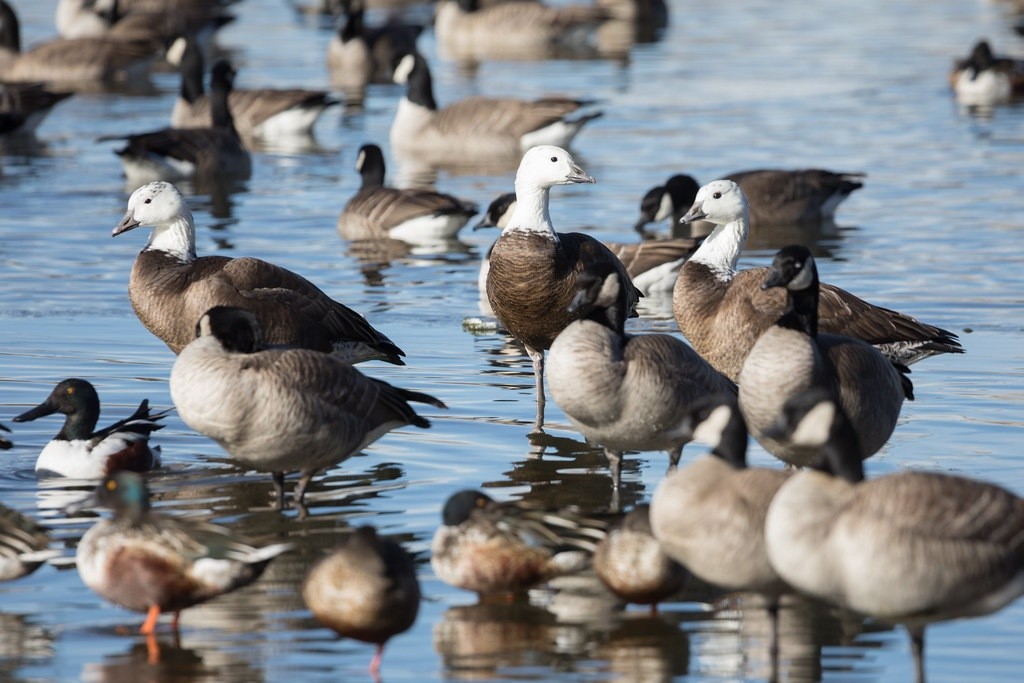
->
[337,145,480,248]
[10,378,175,479]
[111,180,407,367]
[543,232,741,487]
[756,386,1024,683]
[301,525,421,672]
[486,144,645,450]
[950,42,1024,105]
[167,304,450,515]
[65,470,297,637]
[737,243,915,472]
[470,191,701,316]
[429,491,623,597]
[670,179,966,389]
[648,389,866,637]
[2,1,669,182]
[634,168,869,233]
[593,503,730,617]
[0,497,65,584]
